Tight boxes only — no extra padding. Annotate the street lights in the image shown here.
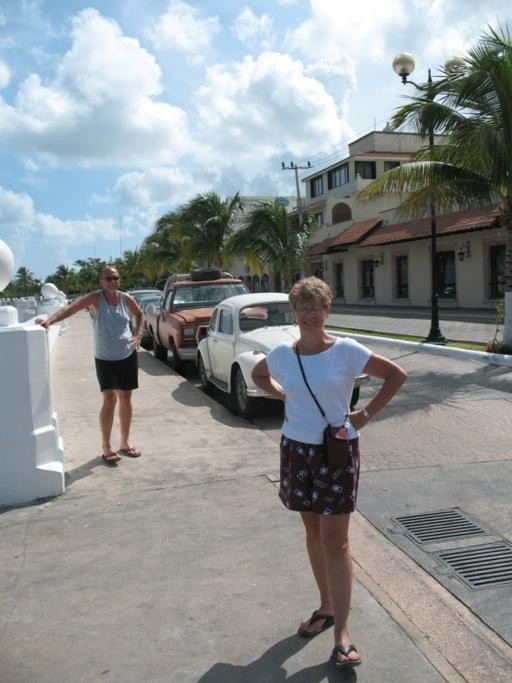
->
[393,53,448,345]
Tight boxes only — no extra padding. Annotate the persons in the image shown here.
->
[35,266,144,464]
[251,275,408,670]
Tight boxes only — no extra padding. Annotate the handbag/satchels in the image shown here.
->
[325,426,351,469]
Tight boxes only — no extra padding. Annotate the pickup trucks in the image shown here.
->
[144,272,267,371]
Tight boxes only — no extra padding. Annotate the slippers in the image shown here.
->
[301,610,334,638]
[120,446,141,457]
[102,451,120,463]
[332,645,361,668]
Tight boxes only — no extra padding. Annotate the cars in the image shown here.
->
[125,289,186,346]
[196,292,369,417]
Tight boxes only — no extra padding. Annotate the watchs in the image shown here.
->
[361,407,372,420]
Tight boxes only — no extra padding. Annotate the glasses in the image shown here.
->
[105,276,119,281]
